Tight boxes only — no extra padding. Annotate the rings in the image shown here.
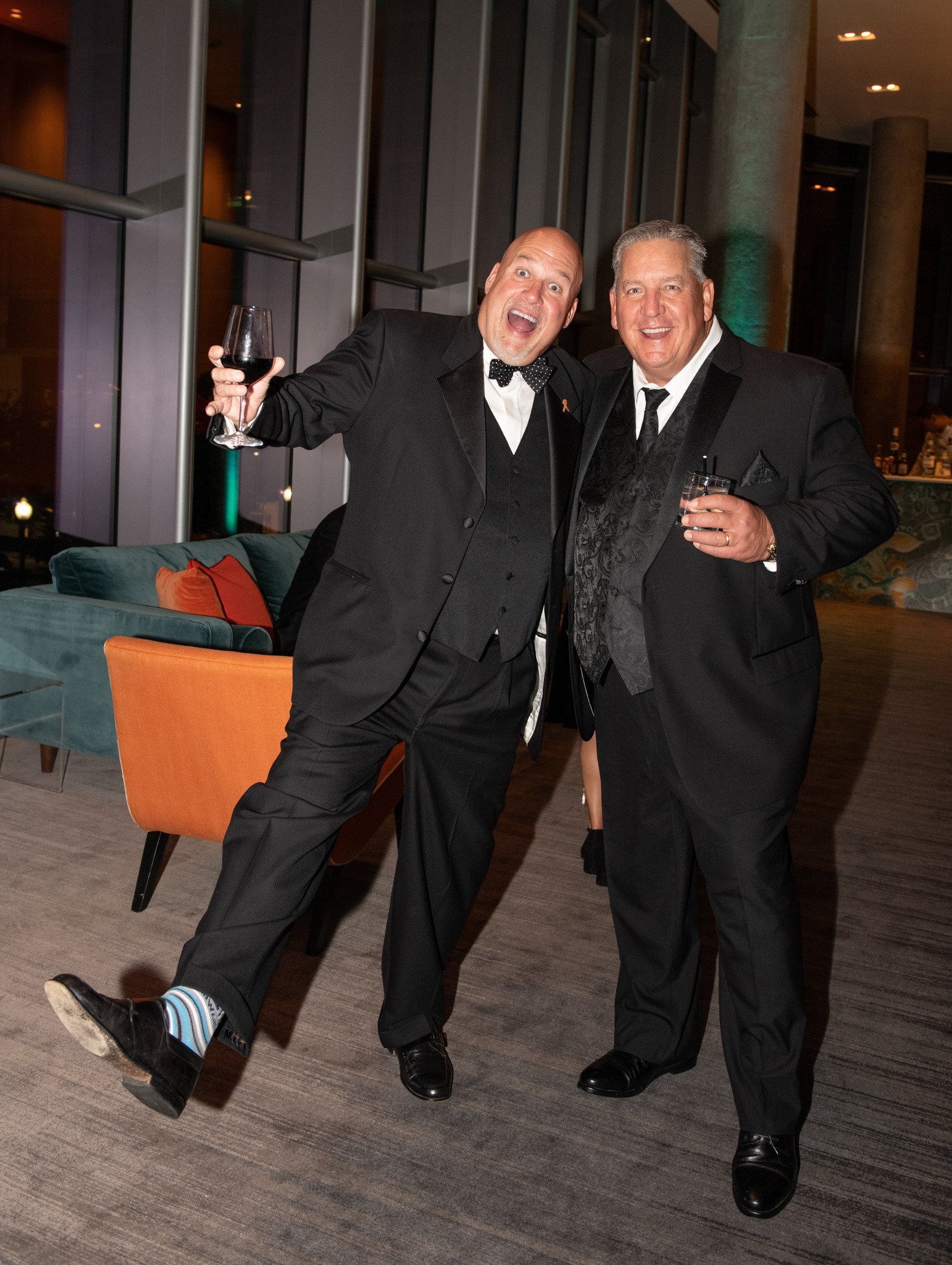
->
[724,531,729,547]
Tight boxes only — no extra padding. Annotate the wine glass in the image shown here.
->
[214,305,274,446]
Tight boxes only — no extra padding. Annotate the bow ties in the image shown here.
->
[487,356,555,395]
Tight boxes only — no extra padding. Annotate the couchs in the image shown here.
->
[1,527,315,776]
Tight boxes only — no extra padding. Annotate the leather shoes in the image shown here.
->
[731,1129,801,1220]
[577,1048,697,1098]
[43,973,206,1121]
[386,1029,454,1102]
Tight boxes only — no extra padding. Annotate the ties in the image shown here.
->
[635,387,670,468]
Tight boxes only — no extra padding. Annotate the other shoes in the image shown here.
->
[595,853,608,887]
[580,826,604,875]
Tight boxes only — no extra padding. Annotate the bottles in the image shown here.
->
[874,425,937,477]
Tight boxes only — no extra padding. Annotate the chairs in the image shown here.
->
[103,636,416,959]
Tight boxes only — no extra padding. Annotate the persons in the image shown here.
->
[571,213,899,1219]
[275,504,403,855]
[910,430,940,475]
[546,593,611,888]
[915,403,952,461]
[40,225,594,1120]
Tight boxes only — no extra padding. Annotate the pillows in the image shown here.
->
[156,566,226,621]
[186,554,276,638]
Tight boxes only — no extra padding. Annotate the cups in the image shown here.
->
[676,471,731,535]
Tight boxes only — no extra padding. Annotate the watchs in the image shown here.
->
[764,543,776,563]
